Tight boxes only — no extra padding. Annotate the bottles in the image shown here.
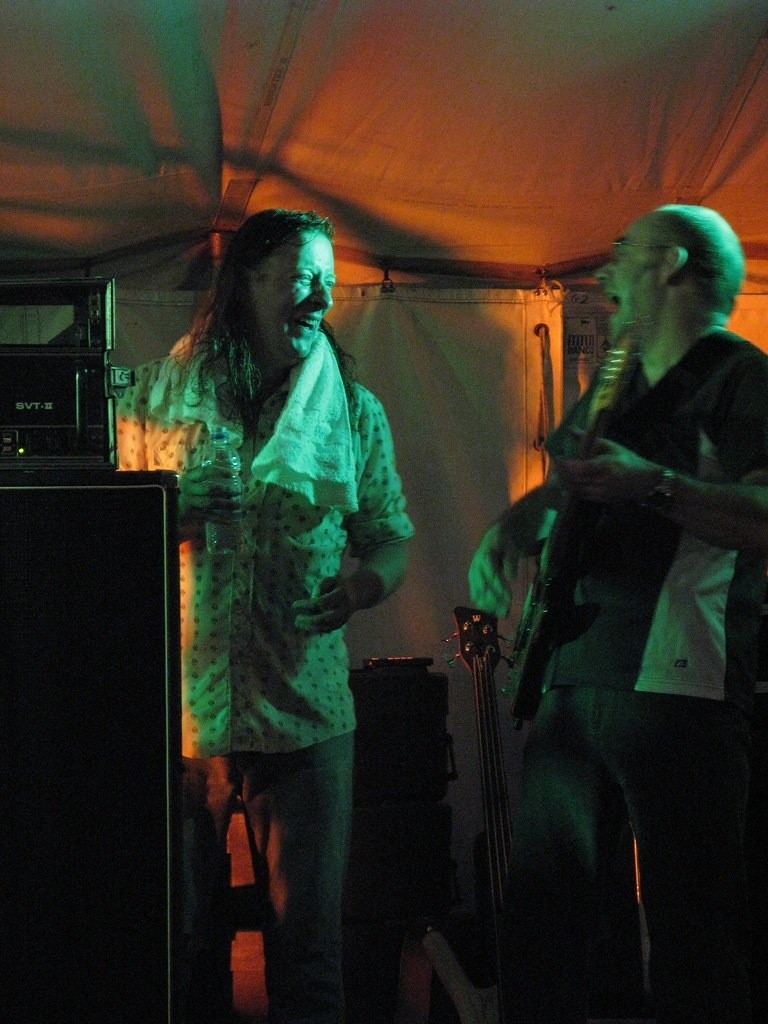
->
[201,425,246,555]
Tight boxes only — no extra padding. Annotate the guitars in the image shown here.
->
[432,607,521,1024]
[501,314,655,721]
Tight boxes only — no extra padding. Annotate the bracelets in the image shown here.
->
[638,465,683,510]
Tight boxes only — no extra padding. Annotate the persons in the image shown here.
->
[113,208,417,1024]
[469,205,768,1024]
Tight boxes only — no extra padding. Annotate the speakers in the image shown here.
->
[0,468,180,1024]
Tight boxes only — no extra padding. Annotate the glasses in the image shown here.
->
[612,237,672,266]
